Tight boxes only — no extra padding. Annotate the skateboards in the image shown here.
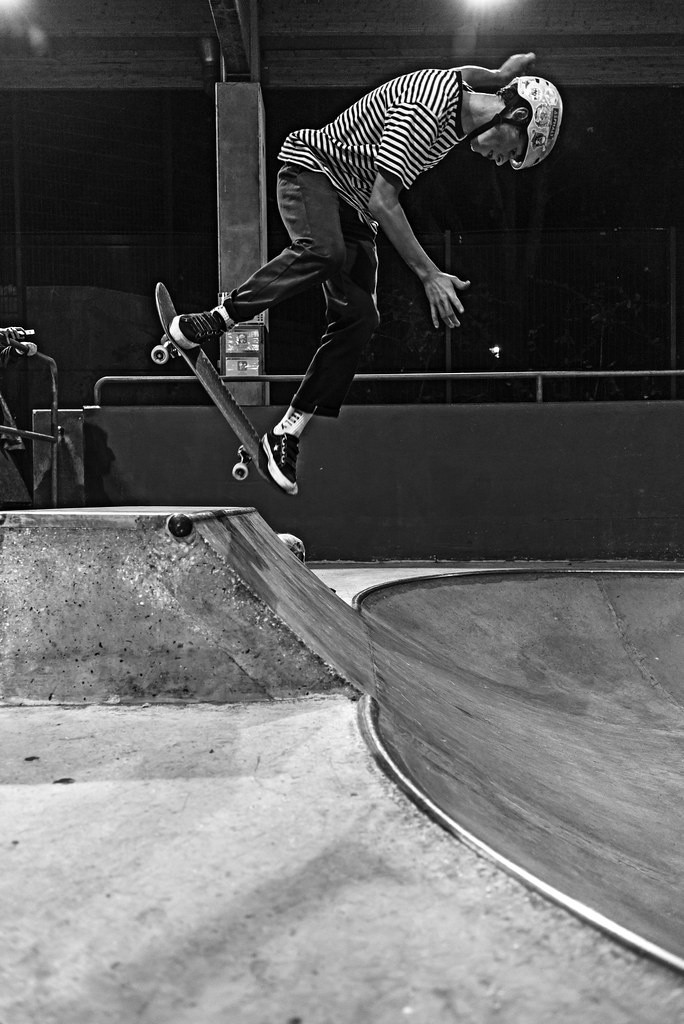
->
[151,282,274,485]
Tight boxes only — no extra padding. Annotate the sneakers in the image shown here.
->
[169,312,227,350]
[261,428,300,491]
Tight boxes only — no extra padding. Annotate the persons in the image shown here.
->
[169,51,563,495]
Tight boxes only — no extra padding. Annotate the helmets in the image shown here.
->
[507,76,563,170]
